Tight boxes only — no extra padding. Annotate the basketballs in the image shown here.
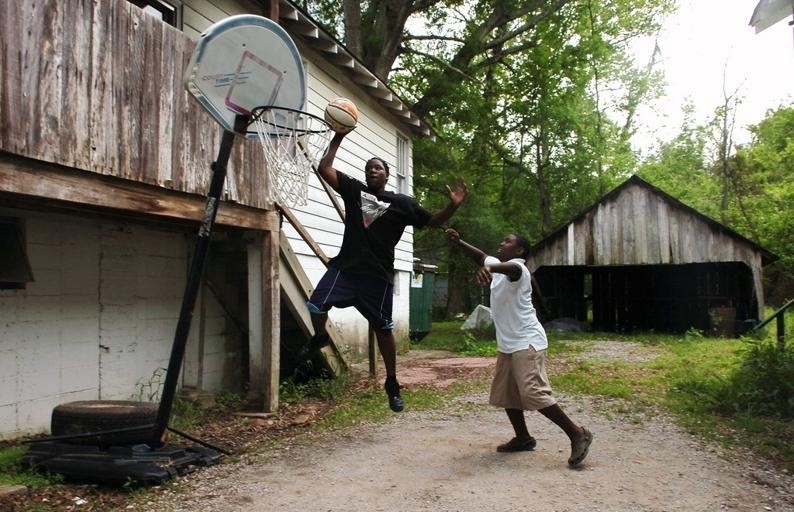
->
[324,97,359,134]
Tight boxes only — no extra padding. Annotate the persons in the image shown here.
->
[444,223,592,467]
[297,123,468,413]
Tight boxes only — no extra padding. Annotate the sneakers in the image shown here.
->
[384,378,404,412]
[497,436,536,452]
[568,425,593,465]
[297,333,331,360]
